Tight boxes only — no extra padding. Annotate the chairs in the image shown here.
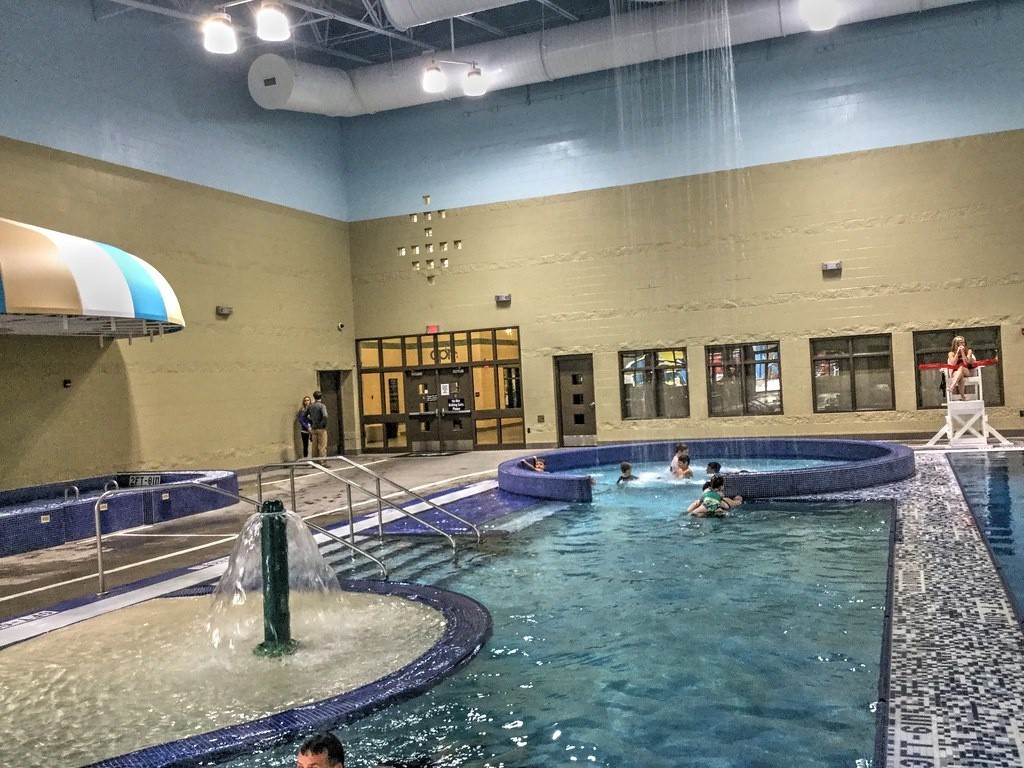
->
[940,362,985,402]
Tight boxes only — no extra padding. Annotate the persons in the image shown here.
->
[298,395,312,459]
[616,462,638,484]
[706,462,721,473]
[693,475,725,513]
[297,732,344,768]
[687,481,742,511]
[670,444,690,472]
[674,454,693,479]
[520,455,546,472]
[947,335,975,401]
[302,391,332,469]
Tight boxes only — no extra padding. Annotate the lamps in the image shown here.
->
[256,0,290,42]
[201,6,238,54]
[420,56,446,93]
[463,59,487,96]
